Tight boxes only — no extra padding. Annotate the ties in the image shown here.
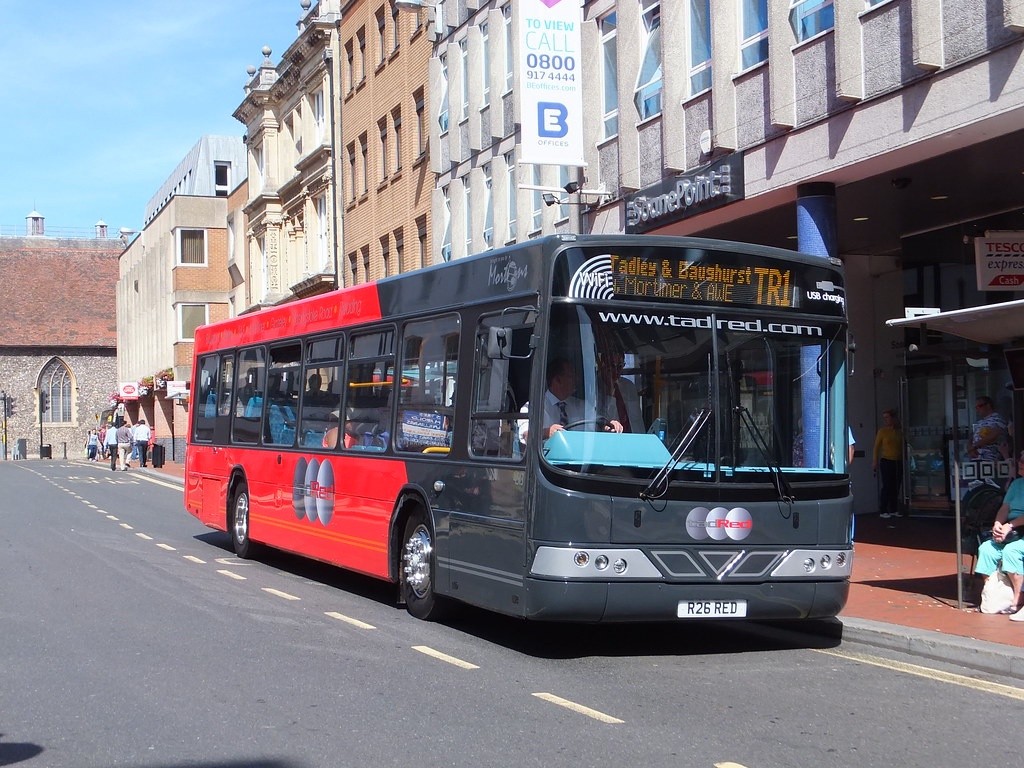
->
[614,382,632,434]
[559,402,569,431]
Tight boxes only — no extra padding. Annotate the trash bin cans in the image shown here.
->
[152,443,165,468]
[40,444,52,459]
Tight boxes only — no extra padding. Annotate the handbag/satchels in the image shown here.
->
[980,560,1014,615]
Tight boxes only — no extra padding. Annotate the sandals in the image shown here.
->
[1001,601,1024,613]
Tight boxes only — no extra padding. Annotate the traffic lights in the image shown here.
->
[6,396,17,418]
[41,391,50,413]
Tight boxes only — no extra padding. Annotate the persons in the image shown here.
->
[974,449,1024,622]
[831,426,856,465]
[792,418,804,467]
[134,419,150,467]
[518,366,623,446]
[117,420,133,471]
[87,425,107,462]
[598,347,645,433]
[872,409,902,528]
[322,398,362,448]
[104,422,119,471]
[972,396,1010,490]
[125,421,140,461]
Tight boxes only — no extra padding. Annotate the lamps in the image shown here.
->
[543,194,560,206]
[564,182,581,194]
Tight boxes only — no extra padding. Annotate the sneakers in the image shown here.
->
[879,511,891,518]
[889,511,903,517]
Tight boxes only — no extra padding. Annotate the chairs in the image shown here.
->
[205,392,387,453]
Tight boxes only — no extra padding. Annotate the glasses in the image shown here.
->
[609,361,626,369]
[975,403,986,409]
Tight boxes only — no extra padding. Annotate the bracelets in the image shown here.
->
[1007,522,1013,528]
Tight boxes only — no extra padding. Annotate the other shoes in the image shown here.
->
[1009,606,1024,622]
[110,463,147,471]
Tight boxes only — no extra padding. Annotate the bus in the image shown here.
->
[183,233,859,624]
